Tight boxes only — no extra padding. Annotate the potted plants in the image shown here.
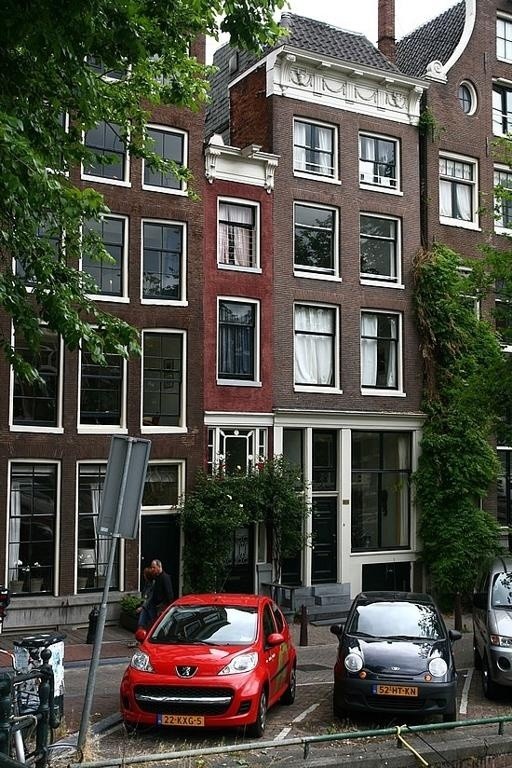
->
[119,592,163,632]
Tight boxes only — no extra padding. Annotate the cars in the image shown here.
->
[124,591,301,740]
[325,588,465,724]
[17,478,99,580]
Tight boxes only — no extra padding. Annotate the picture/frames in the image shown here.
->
[163,357,175,388]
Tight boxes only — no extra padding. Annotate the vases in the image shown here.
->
[9,580,25,592]
[94,575,107,588]
[27,578,44,592]
[78,576,89,588]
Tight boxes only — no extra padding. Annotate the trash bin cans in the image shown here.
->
[12,632,67,729]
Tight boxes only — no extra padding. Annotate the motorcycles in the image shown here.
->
[0,585,9,615]
[0,629,66,767]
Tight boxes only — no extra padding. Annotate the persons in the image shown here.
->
[128,567,155,648]
[151,559,173,627]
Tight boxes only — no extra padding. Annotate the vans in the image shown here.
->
[469,551,512,706]
